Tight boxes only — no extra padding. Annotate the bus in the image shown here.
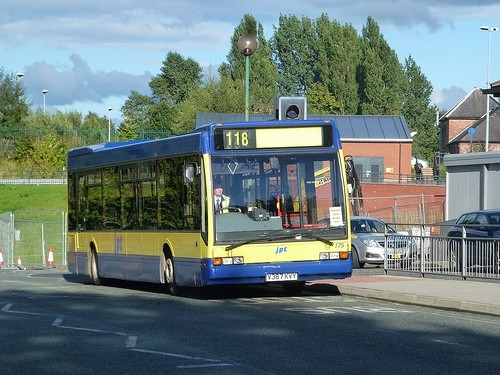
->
[65,119,358,297]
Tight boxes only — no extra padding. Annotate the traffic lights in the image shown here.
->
[280,98,308,121]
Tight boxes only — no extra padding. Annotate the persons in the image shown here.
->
[213,182,233,214]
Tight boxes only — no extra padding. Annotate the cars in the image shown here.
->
[317,216,417,270]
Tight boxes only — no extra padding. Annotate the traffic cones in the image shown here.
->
[0,249,5,268]
[46,244,55,269]
[17,256,22,270]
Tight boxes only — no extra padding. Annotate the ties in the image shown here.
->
[216,199,220,210]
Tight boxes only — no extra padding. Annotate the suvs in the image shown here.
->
[446,209,500,275]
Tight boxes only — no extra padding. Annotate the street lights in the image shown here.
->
[479,26,498,152]
[108,108,113,142]
[17,73,25,102]
[42,89,49,114]
[237,34,258,122]
